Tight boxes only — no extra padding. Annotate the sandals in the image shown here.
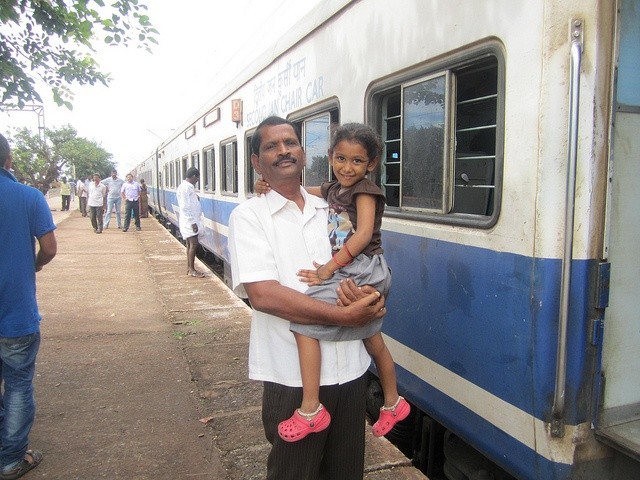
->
[372,396,410,437]
[0,449,44,480]
[278,404,331,443]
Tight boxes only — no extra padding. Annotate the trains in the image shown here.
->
[124,0,639,480]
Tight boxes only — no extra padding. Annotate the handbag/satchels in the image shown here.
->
[77,189,83,199]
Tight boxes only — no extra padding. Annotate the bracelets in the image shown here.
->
[334,255,349,266]
[316,265,335,280]
[343,244,354,259]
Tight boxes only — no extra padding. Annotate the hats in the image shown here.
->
[79,177,85,180]
[61,177,66,179]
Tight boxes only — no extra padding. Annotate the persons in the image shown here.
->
[1,133,57,479]
[54,169,149,234]
[176,168,205,279]
[225,115,411,479]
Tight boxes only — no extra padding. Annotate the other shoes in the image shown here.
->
[61,209,65,211]
[136,226,141,231]
[122,228,127,232]
[93,226,98,232]
[95,230,102,234]
[186,269,205,278]
